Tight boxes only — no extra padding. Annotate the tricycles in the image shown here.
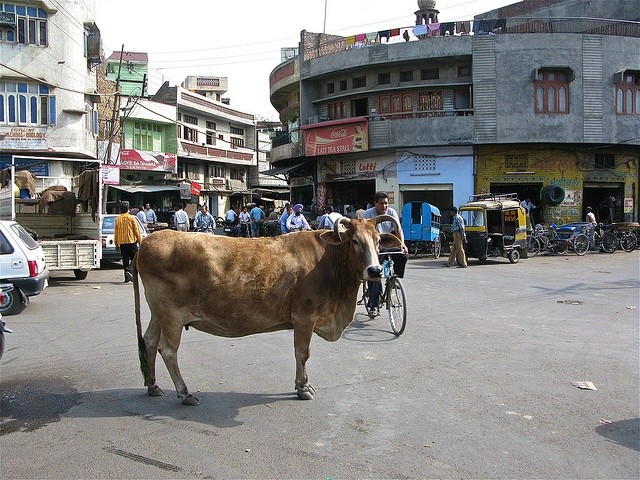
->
[402,201,442,257]
[458,193,526,263]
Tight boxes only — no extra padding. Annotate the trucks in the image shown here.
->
[1,155,103,281]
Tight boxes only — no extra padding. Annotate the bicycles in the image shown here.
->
[529,222,637,254]
[361,249,408,335]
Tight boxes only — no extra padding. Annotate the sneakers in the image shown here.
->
[368,307,380,319]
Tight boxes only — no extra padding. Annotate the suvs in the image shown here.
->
[0,221,48,314]
[103,214,146,258]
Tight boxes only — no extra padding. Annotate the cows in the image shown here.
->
[134,214,405,406]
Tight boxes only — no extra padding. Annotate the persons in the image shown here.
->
[113,200,142,282]
[522,207,532,254]
[520,196,540,217]
[323,205,344,230]
[173,202,190,232]
[533,217,546,251]
[356,205,366,219]
[136,205,147,224]
[441,206,469,268]
[318,208,326,229]
[250,202,266,237]
[145,204,158,223]
[585,206,597,224]
[362,192,410,317]
[225,206,238,223]
[351,123,367,151]
[285,204,313,231]
[193,205,216,235]
[238,207,253,236]
[268,208,278,220]
[345,206,357,219]
[280,204,294,234]
